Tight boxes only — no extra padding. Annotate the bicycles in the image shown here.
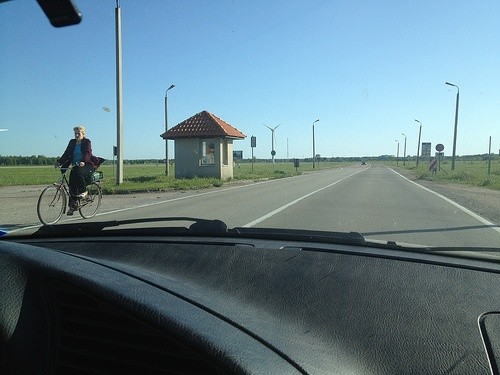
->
[37,164,103,225]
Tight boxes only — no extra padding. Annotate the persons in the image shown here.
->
[54,125,105,216]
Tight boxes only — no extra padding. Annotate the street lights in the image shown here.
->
[415,119,422,167]
[395,139,399,165]
[445,82,459,169]
[401,132,407,164]
[313,119,320,169]
[165,83,175,176]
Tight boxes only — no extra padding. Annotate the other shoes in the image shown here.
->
[67,207,74,215]
[76,191,88,197]
